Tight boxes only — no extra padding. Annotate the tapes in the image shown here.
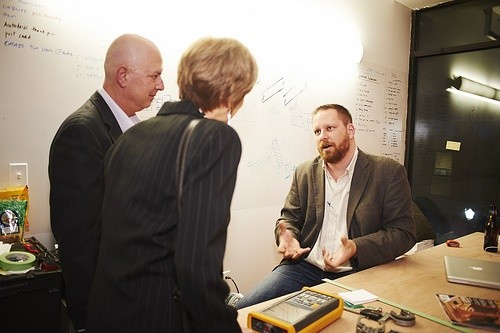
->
[0,251,36,272]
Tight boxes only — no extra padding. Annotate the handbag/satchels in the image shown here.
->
[484,205,498,253]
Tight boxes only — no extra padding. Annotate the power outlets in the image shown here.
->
[223,270,231,285]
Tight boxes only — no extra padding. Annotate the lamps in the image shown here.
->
[449,76,500,101]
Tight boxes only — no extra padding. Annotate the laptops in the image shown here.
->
[444,255,500,289]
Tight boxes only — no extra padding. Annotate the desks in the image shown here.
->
[0,234,65,333]
[234,232,500,333]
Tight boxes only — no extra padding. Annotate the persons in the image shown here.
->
[89,37,259,333]
[234,104,417,311]
[48,33,164,333]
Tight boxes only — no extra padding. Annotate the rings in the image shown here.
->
[331,259,334,262]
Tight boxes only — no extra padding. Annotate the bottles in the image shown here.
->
[483,205,499,252]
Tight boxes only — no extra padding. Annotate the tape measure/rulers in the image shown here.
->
[322,277,473,333]
